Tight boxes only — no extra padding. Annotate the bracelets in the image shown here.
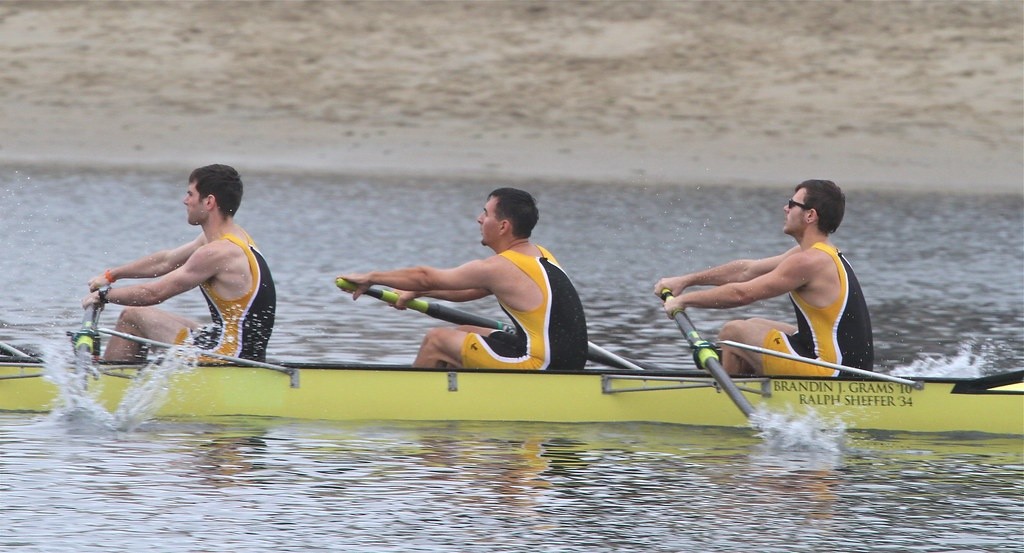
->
[105,269,116,283]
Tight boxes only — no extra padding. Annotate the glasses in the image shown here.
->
[789,198,813,211]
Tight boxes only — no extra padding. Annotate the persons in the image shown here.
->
[80,164,276,368]
[334,188,589,371]
[655,179,874,377]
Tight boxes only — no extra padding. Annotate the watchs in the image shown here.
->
[98,286,111,303]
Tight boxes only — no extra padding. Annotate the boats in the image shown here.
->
[1,349,1024,431]
